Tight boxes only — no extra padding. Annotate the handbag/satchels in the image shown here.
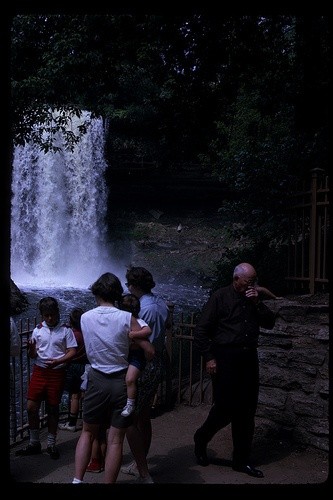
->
[154,302,180,369]
[138,367,172,417]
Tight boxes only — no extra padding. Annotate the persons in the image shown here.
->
[58,309,91,432]
[121,267,169,484]
[85,426,107,473]
[15,297,79,460]
[194,262,276,477]
[117,293,152,417]
[72,273,155,484]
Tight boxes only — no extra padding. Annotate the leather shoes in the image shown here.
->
[232,462,264,478]
[194,431,208,466]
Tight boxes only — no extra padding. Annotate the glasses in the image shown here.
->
[125,282,131,287]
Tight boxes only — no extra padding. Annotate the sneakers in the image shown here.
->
[58,422,76,432]
[121,405,136,417]
[47,445,60,460]
[15,444,41,457]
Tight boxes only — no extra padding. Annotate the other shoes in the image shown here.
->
[140,477,155,484]
[84,457,104,473]
[120,463,140,479]
